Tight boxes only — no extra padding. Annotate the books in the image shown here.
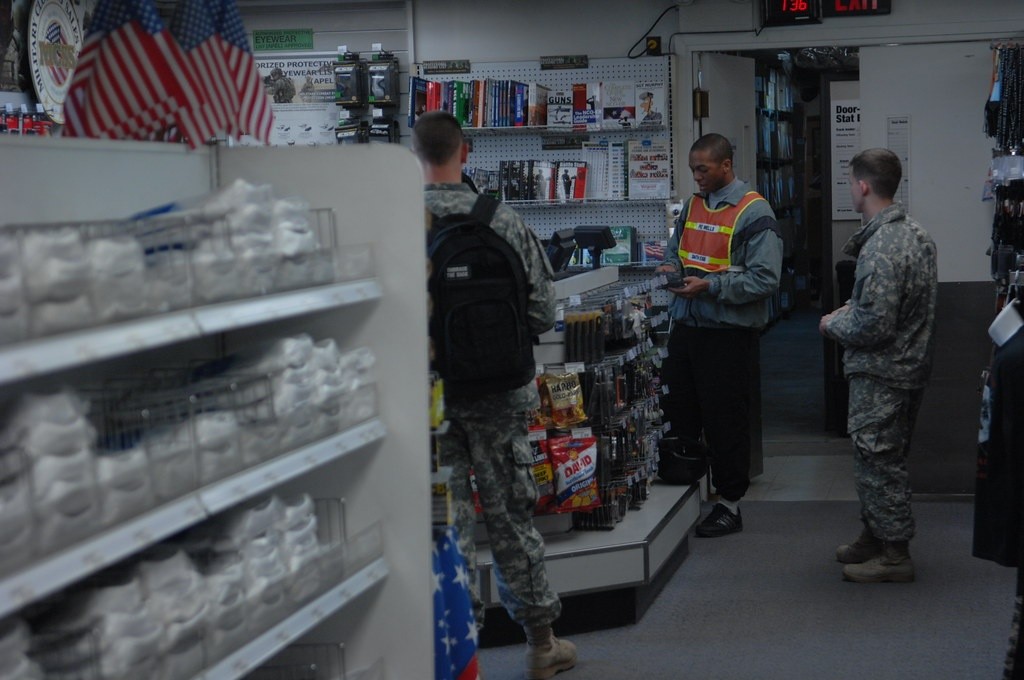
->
[462,139,670,200]
[567,226,667,267]
[409,77,665,128]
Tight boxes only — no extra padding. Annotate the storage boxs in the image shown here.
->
[603,226,637,264]
[568,239,581,265]
[582,249,603,267]
[640,241,668,267]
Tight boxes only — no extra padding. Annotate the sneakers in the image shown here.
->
[696,502,743,537]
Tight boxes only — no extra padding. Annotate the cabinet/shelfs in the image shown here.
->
[411,54,681,281]
[0,136,432,680]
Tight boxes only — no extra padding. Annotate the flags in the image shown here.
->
[61,0,274,150]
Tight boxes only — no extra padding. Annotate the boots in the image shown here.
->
[837,528,883,562]
[522,624,578,680]
[843,537,914,585]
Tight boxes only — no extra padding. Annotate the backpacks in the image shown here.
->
[427,193,537,393]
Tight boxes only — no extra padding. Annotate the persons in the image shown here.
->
[818,147,939,584]
[562,169,573,203]
[655,132,785,539]
[535,166,548,202]
[264,67,293,103]
[413,111,578,680]
